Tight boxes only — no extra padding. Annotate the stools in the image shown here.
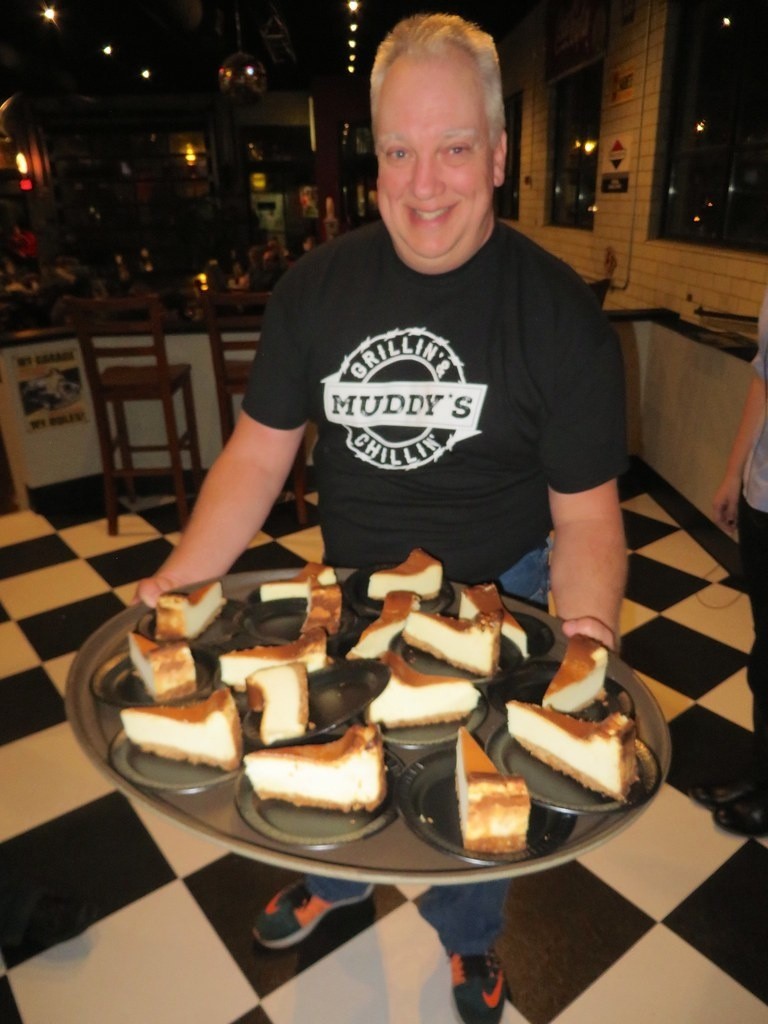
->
[206,292,315,529]
[65,295,201,536]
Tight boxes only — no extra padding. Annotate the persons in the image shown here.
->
[0,222,298,320]
[131,13,629,1024]
[688,296,768,833]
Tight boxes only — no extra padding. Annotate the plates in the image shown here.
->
[505,610,556,661]
[239,657,388,750]
[233,734,408,850]
[234,597,360,647]
[391,745,579,867]
[88,639,227,707]
[108,727,258,795]
[483,719,664,815]
[367,696,487,752]
[341,562,455,619]
[137,597,246,646]
[485,660,635,725]
[388,628,522,685]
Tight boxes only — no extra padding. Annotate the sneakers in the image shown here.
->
[252,881,375,947]
[448,947,506,1024]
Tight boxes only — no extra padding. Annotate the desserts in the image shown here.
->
[118,548,638,855]
